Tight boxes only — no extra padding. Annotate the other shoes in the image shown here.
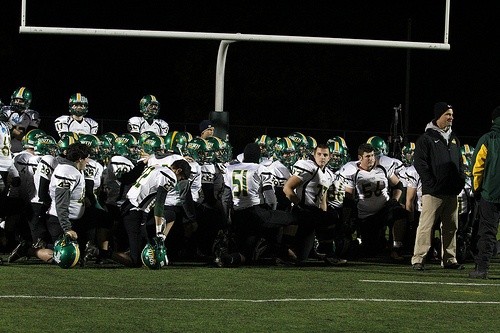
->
[83,241,99,263]
[323,257,347,266]
[444,264,466,270]
[213,246,225,268]
[412,263,425,271]
[469,264,488,279]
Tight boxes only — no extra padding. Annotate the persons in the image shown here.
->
[470,109,499,278]
[0,87,500,269]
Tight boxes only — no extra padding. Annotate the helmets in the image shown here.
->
[53,234,81,269]
[402,143,414,164]
[11,87,32,105]
[20,129,233,162]
[69,93,88,116]
[460,144,475,176]
[139,95,160,113]
[254,132,317,167]
[366,136,384,153]
[327,136,348,170]
[141,237,166,270]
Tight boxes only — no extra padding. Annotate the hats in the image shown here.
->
[200,120,215,133]
[434,101,453,121]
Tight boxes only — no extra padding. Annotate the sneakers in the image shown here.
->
[8,239,28,263]
